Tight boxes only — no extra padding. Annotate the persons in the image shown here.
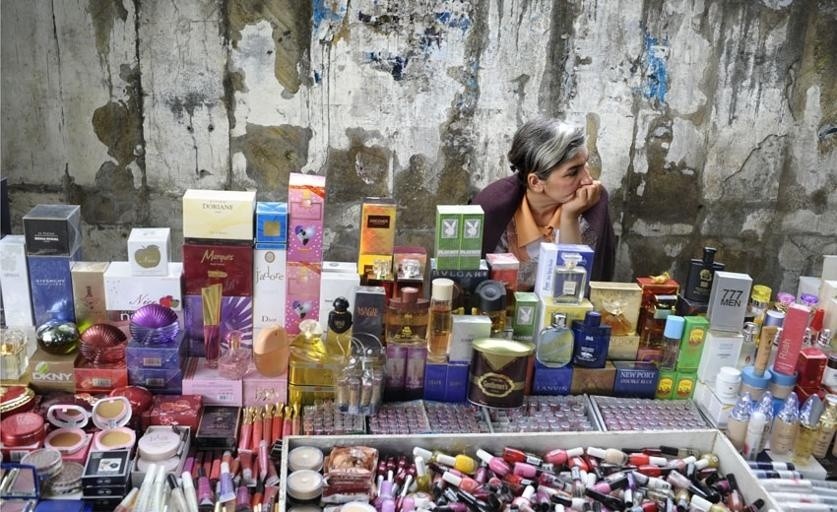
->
[467,115,615,292]
